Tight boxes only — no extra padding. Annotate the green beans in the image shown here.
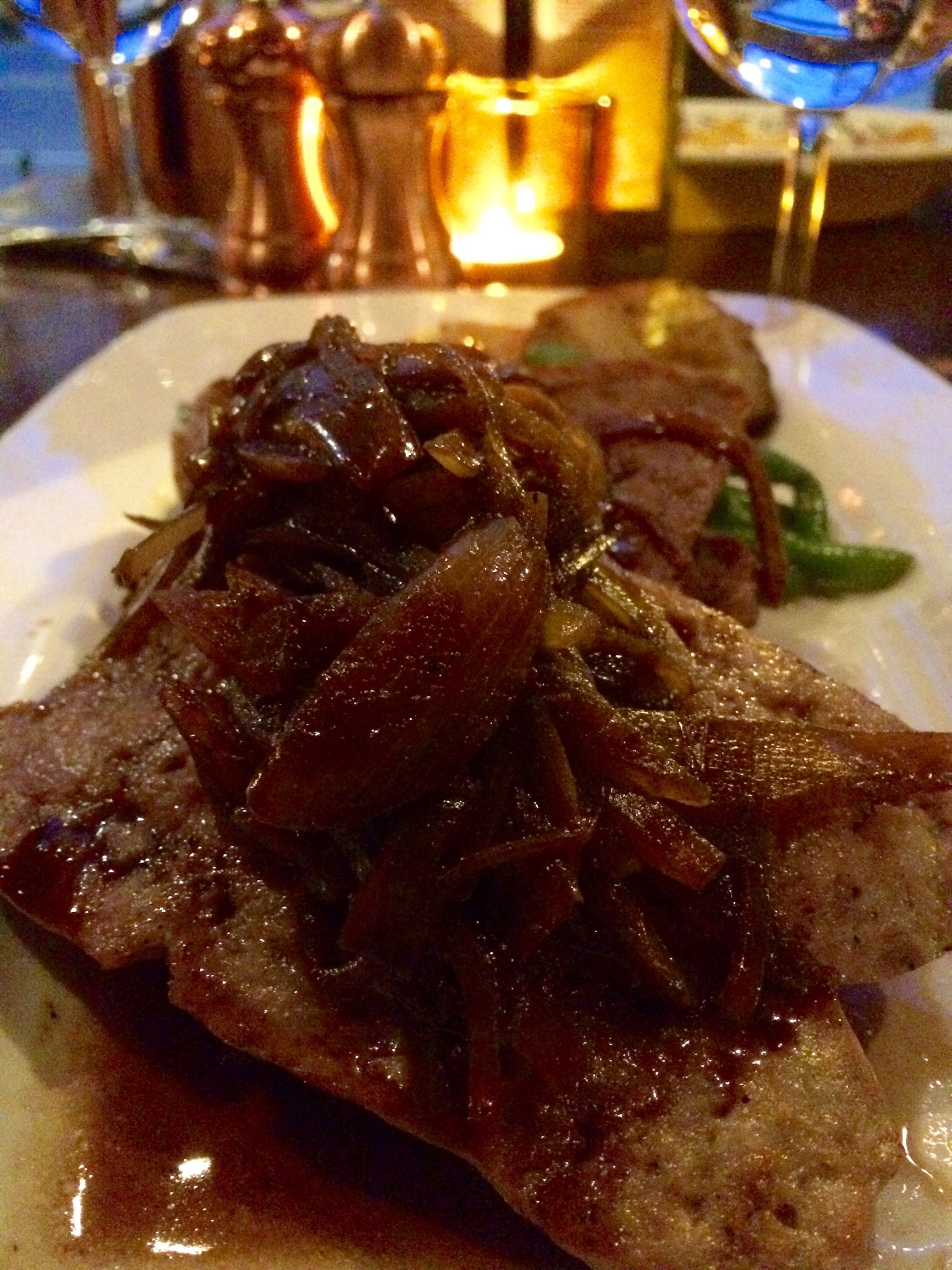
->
[520,338,914,591]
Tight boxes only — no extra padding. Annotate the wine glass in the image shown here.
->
[668,0,952,322]
[1,1,228,268]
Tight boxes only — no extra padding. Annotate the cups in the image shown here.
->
[432,77,609,281]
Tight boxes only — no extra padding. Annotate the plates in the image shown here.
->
[670,95,952,227]
[1,283,952,1270]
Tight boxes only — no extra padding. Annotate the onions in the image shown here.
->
[611,701,952,816]
[111,312,790,1115]
